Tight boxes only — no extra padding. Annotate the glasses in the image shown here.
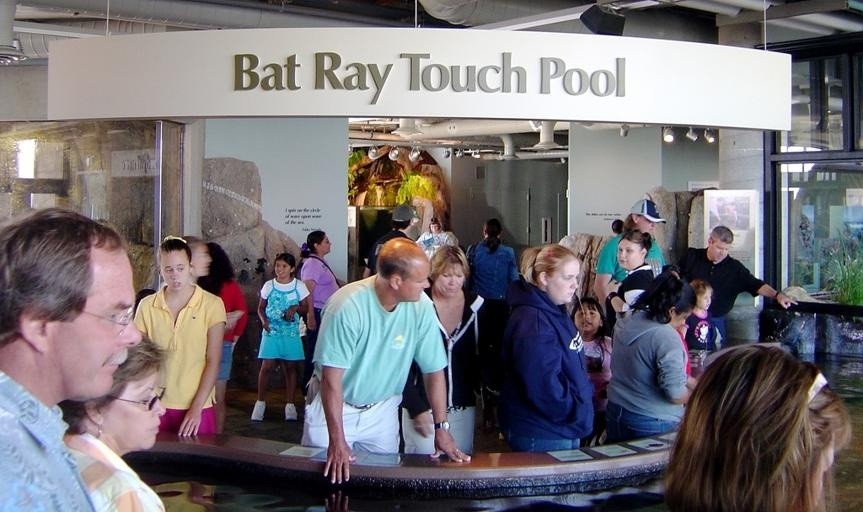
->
[82,310,135,338]
[107,386,165,410]
[801,362,829,404]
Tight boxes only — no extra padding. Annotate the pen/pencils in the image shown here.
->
[649,443,665,446]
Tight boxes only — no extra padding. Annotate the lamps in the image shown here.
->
[575,0,631,37]
[442,145,464,159]
[367,145,424,163]
[619,125,716,143]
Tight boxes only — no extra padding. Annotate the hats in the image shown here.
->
[392,205,413,222]
[631,199,667,223]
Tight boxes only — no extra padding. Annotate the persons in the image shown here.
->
[322,489,351,512]
[150,479,220,512]
[135,197,800,486]
[663,344,857,512]
[57,329,173,511]
[1,205,143,511]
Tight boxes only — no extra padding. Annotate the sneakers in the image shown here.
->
[285,403,297,420]
[251,400,265,420]
